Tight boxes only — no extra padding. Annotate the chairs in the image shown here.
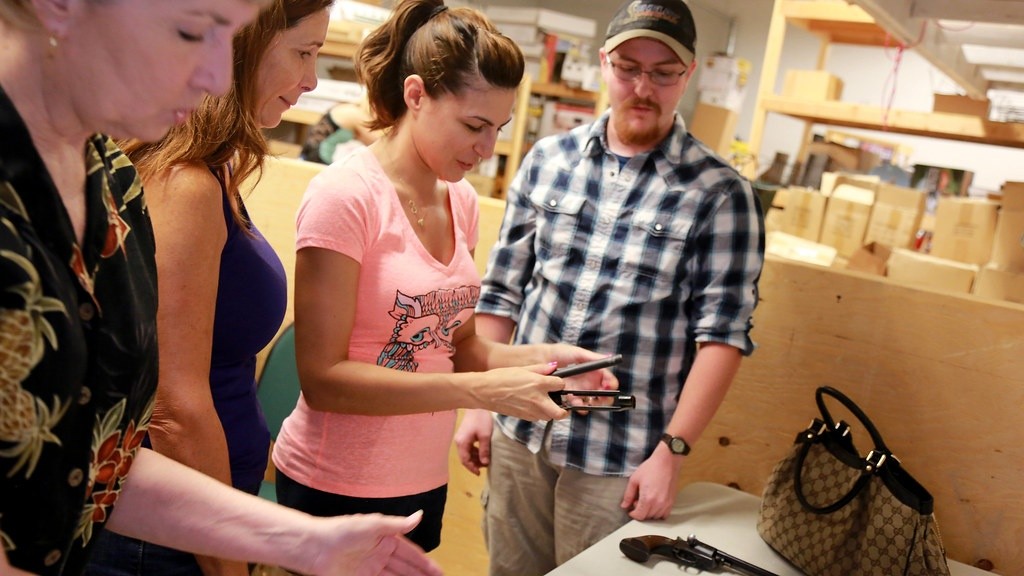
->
[255,321,302,504]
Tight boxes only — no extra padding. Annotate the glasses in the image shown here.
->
[610,61,688,87]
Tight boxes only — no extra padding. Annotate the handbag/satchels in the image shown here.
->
[757,385,951,576]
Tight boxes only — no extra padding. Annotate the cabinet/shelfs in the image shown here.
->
[259,0,1024,203]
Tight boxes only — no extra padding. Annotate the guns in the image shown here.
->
[618,534,778,576]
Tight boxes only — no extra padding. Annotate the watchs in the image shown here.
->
[659,433,692,456]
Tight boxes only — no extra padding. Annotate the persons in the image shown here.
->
[453,0,768,576]
[122,2,330,576]
[301,103,390,168]
[269,0,619,576]
[0,1,442,576]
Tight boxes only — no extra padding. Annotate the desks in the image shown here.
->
[544,480,1002,576]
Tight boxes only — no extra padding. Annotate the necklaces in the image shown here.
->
[394,169,438,227]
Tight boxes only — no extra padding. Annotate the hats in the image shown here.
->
[604,0,697,67]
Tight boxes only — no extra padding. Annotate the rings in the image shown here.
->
[583,396,597,402]
